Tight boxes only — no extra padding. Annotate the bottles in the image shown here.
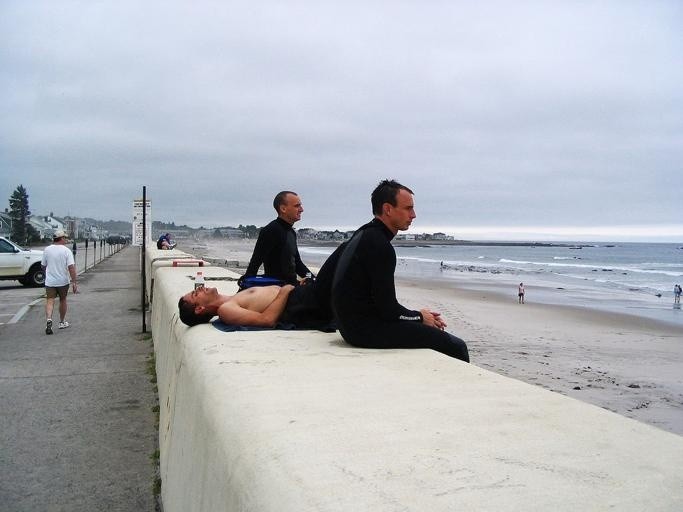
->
[194,271,205,290]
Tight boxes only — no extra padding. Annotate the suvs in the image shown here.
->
[0,235,46,288]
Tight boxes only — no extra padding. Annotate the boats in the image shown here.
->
[569,247,582,249]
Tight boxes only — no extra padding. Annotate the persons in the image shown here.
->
[673,284,680,303]
[518,282,524,304]
[237,191,316,293]
[332,178,470,363]
[677,285,682,303]
[178,242,346,333]
[40,230,79,335]
[156,233,176,250]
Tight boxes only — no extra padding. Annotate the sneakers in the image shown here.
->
[59,321,70,329]
[45,319,53,334]
[168,243,177,250]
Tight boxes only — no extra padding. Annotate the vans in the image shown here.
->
[106,236,126,245]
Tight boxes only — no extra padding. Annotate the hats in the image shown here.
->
[53,231,68,239]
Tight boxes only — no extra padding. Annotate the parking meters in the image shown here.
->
[71,238,125,272]
